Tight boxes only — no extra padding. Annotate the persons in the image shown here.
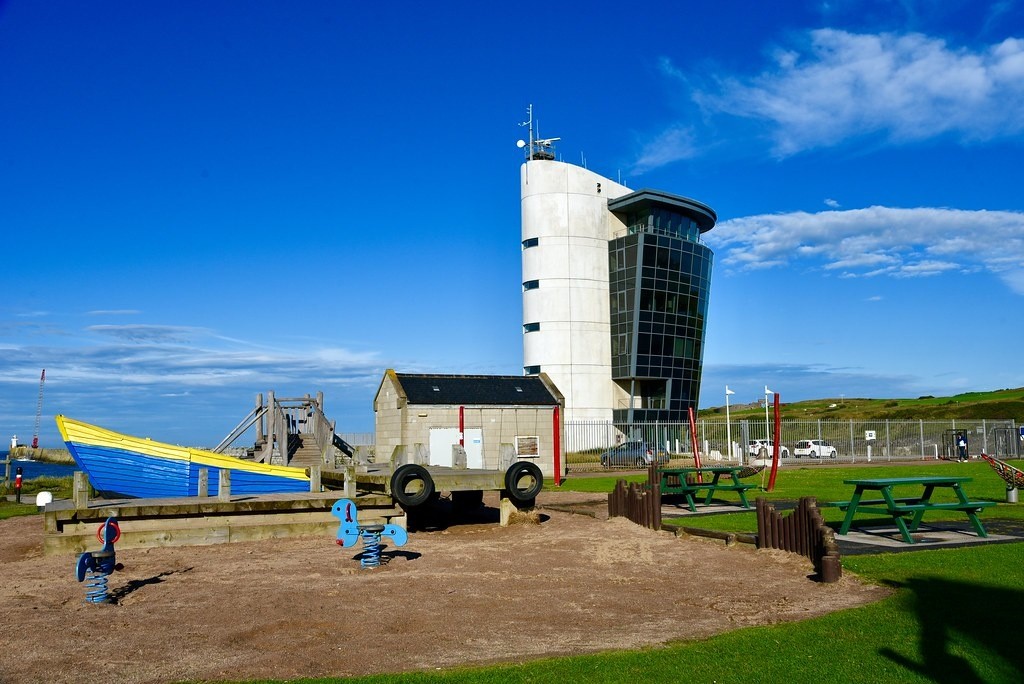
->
[955,432,968,462]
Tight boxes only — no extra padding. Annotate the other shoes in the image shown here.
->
[963,460,968,462]
[957,460,961,463]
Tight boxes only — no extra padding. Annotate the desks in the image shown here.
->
[838,476,988,544]
[656,467,750,512]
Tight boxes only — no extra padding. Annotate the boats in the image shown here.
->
[52,414,325,499]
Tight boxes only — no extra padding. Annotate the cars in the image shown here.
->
[794,439,837,458]
[748,438,789,459]
[600,441,670,469]
[829,404,837,407]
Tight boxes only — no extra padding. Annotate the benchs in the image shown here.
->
[816,498,922,506]
[887,502,995,512]
[681,484,758,490]
[643,482,714,488]
